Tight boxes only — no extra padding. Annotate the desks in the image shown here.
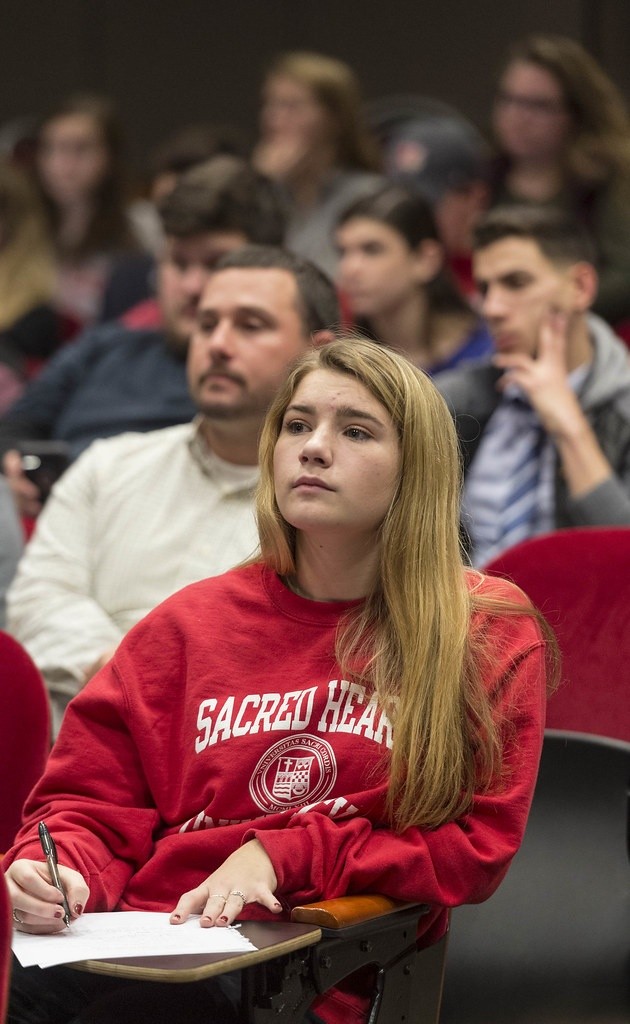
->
[57,910,323,1024]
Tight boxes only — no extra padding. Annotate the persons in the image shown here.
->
[6,246,342,749]
[0,30,630,575]
[0,337,560,1024]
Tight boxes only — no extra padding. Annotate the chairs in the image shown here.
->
[0,526,629,1024]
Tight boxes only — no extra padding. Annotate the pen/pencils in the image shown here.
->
[38,820,71,927]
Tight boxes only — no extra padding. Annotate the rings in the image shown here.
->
[12,910,21,922]
[230,891,246,905]
[209,895,227,903]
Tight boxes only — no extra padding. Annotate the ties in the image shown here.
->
[497,414,541,554]
